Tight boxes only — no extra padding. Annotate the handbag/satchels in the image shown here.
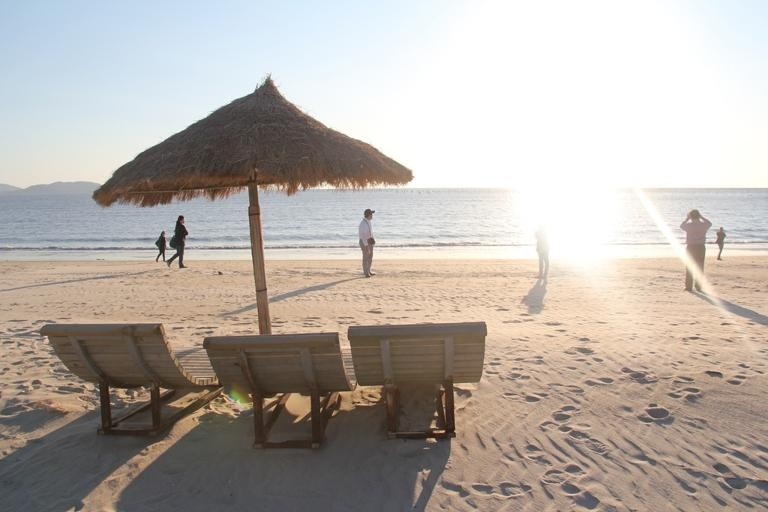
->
[155,240,159,246]
[369,238,375,244]
[170,235,177,248]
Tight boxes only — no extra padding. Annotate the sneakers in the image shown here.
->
[365,272,375,277]
[180,265,187,268]
[167,259,170,267]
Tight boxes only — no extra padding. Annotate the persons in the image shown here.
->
[680,210,713,291]
[167,215,188,268]
[359,209,375,278]
[155,231,166,263]
[534,227,549,279]
[715,227,726,260]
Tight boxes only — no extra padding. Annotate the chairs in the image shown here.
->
[203,334,357,451]
[348,321,487,441]
[40,325,223,438]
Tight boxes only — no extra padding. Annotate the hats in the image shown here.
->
[364,209,375,215]
